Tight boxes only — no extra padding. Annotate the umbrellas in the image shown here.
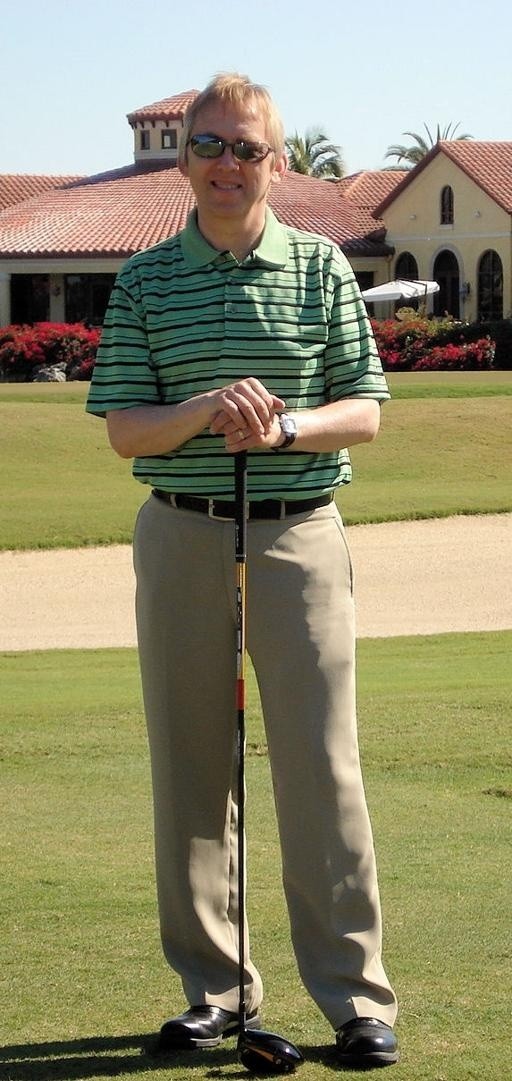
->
[361,276,440,311]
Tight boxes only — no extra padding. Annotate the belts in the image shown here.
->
[153,485,334,523]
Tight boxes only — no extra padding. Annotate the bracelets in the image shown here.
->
[274,410,298,455]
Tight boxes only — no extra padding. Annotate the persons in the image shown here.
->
[81,66,402,1070]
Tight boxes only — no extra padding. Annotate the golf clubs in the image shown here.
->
[232,447,305,1075]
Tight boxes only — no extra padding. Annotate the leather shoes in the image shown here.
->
[160,1003,260,1048]
[336,1016,399,1063]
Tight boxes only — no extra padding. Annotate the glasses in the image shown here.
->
[186,133,276,161]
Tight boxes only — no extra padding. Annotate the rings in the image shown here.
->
[238,430,245,440]
